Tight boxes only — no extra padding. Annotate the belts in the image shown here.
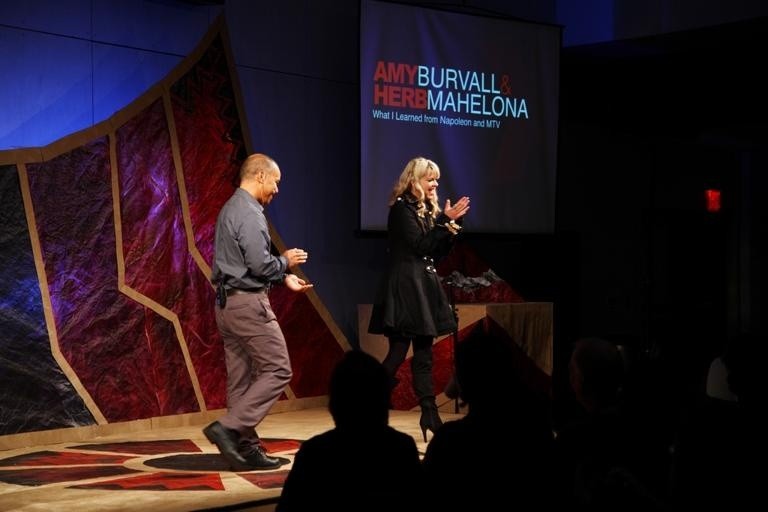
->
[226,286,268,297]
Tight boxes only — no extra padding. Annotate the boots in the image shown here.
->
[410,353,444,441]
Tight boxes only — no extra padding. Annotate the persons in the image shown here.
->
[203,154,314,472]
[368,157,470,442]
[274,333,768,512]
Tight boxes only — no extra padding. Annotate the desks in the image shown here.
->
[355,301,554,414]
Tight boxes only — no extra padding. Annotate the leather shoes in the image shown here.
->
[202,420,253,471]
[239,440,281,470]
